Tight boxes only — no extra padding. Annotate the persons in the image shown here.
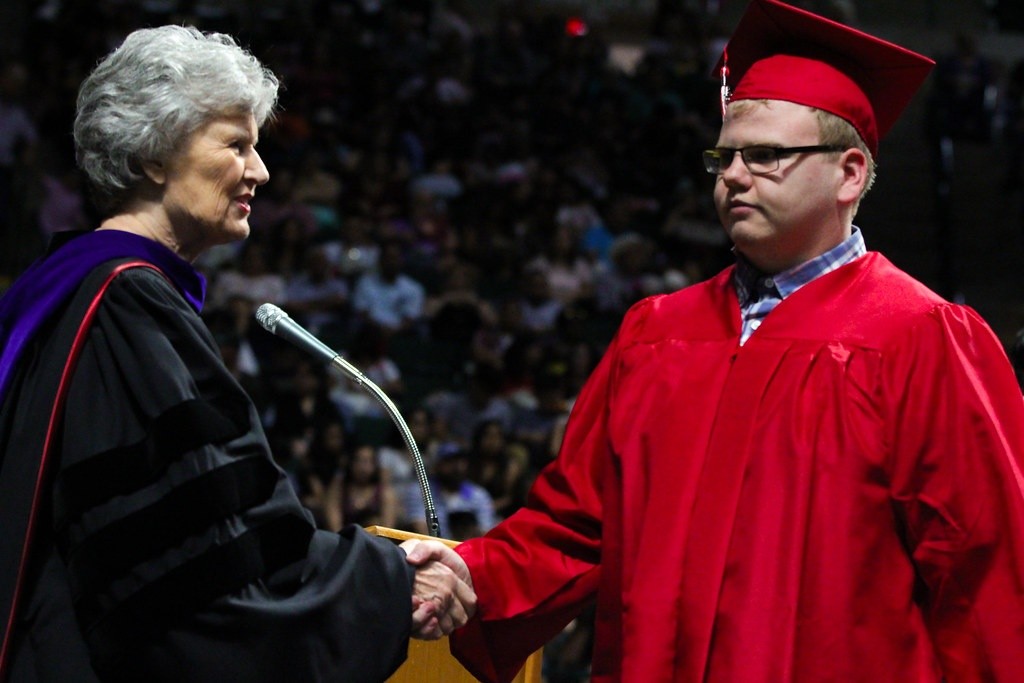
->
[0,0,1024,549]
[396,0,1023,683]
[0,22,477,683]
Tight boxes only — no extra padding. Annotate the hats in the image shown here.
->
[706,2,937,161]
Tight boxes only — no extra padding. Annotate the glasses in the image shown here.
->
[701,143,850,175]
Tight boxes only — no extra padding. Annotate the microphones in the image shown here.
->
[255,303,441,538]
